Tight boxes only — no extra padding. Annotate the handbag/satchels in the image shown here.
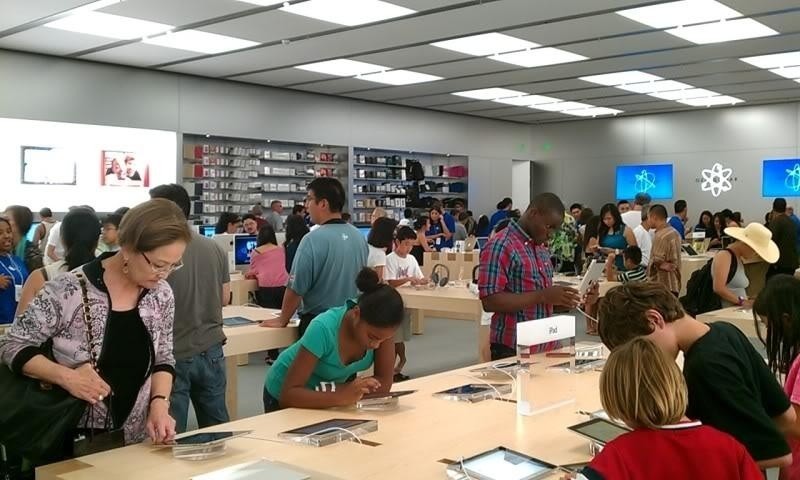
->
[24,241,42,271]
[679,258,723,314]
[0,344,90,466]
[406,159,424,181]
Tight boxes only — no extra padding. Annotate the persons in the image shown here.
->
[123,156,141,180]
[106,158,122,180]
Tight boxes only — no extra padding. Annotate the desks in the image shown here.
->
[393,270,625,364]
[697,303,770,340]
[675,248,715,296]
[34,342,686,480]
[224,278,258,367]
[424,249,480,321]
[219,303,303,420]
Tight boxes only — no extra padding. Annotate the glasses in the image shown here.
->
[136,245,185,275]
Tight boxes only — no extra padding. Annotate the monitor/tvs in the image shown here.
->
[199,225,216,239]
[356,225,372,242]
[213,233,249,274]
[762,159,800,198]
[25,222,41,242]
[614,164,673,200]
[234,234,258,271]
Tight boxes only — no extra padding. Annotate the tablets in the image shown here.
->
[361,390,418,400]
[432,383,512,403]
[278,417,377,447]
[576,259,609,304]
[223,317,255,327]
[453,445,557,480]
[154,430,251,447]
[470,361,539,375]
[568,418,633,447]
[550,358,606,374]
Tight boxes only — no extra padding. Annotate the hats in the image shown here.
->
[723,222,781,264]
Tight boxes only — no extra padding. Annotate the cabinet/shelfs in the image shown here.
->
[354,146,406,229]
[261,139,339,222]
[421,153,468,220]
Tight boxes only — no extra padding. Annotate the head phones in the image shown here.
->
[432,264,449,286]
